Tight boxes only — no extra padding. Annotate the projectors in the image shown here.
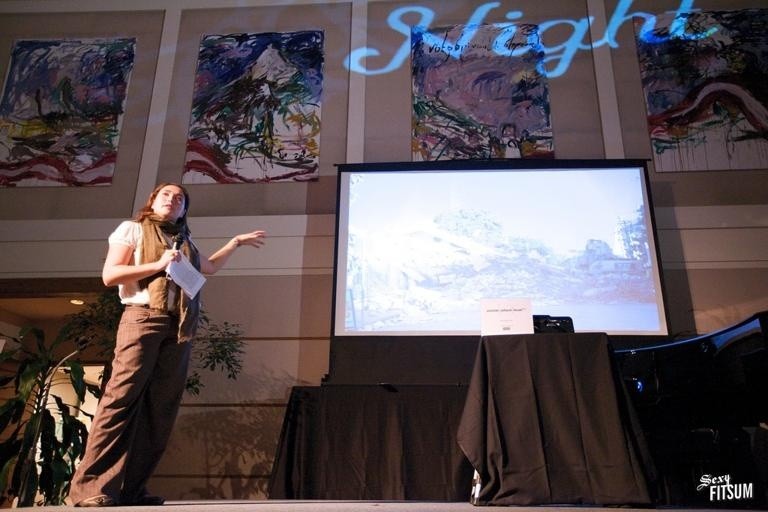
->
[533,313,575,334]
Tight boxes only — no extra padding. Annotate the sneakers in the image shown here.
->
[79,495,113,506]
[137,496,165,505]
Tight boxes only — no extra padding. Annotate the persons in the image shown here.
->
[70,180,266,506]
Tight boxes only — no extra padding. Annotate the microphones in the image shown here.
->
[165,232,183,284]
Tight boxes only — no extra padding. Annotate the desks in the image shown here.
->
[462,334,640,505]
[290,384,479,500]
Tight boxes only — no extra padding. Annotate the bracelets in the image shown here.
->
[233,236,242,247]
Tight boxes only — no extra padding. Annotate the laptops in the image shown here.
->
[480,296,534,337]
